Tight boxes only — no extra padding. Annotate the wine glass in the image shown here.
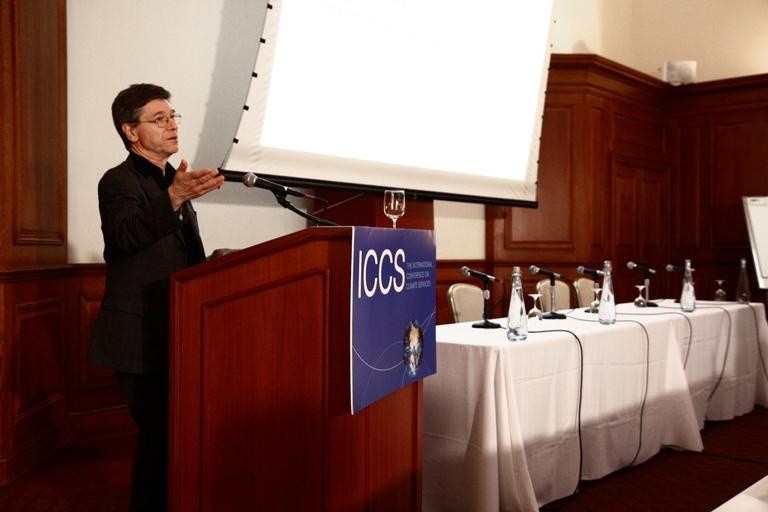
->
[589,288,602,314]
[527,293,543,325]
[634,285,647,310]
[715,280,727,306]
[383,190,405,228]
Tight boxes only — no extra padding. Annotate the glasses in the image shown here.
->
[125,115,183,129]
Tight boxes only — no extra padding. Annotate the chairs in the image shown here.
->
[572,277,599,308]
[536,278,571,311]
[447,283,485,323]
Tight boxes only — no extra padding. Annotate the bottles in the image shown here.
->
[506,266,528,341]
[598,260,616,324]
[680,259,696,312]
[736,259,751,304]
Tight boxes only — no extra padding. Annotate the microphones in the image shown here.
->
[460,265,499,285]
[243,171,314,204]
[627,262,657,275]
[528,265,565,285]
[576,265,603,278]
[666,265,695,274]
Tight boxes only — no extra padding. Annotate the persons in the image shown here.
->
[89,83,228,510]
